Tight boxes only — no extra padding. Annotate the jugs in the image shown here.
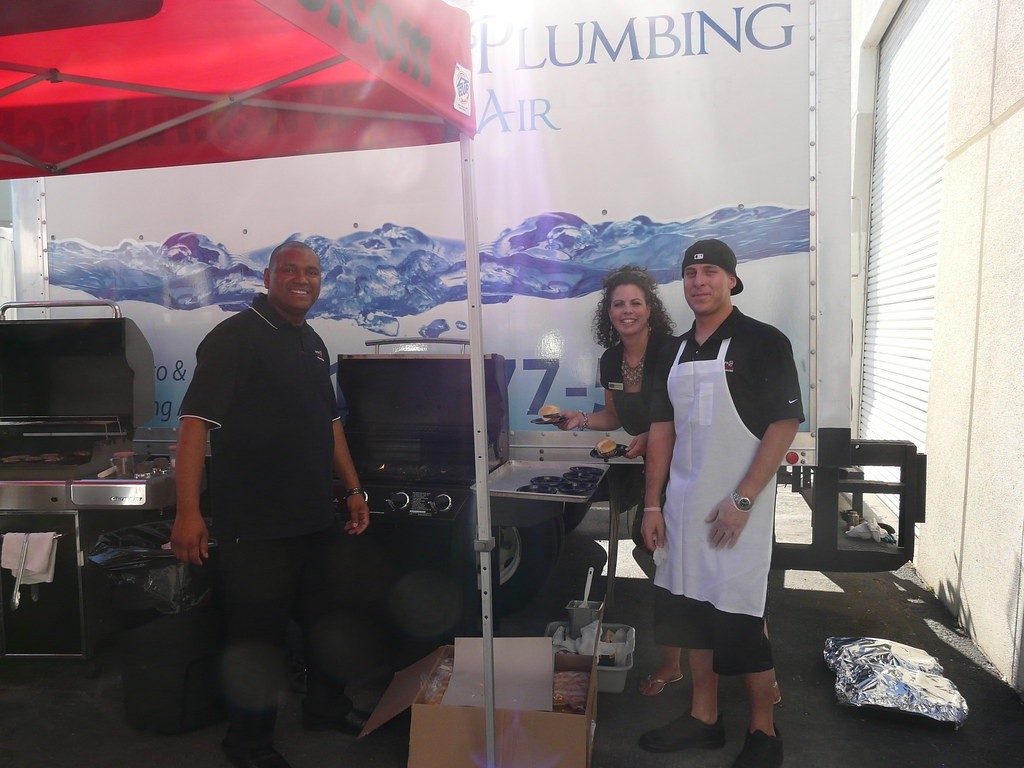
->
[110,452,136,478]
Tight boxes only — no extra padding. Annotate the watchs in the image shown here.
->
[732,491,753,513]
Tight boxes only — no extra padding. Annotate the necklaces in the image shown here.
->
[621,349,646,384]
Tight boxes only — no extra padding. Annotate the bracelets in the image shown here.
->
[578,410,588,431]
[345,488,364,497]
[643,507,661,512]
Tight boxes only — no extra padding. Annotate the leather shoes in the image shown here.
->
[222,736,291,768]
[303,706,369,736]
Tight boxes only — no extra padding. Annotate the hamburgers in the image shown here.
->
[597,439,616,458]
[539,405,562,421]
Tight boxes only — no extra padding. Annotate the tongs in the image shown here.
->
[11,532,40,610]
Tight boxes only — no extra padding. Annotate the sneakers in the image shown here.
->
[732,721,784,768]
[639,712,727,752]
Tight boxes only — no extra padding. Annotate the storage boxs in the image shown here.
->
[545,618,635,694]
[353,645,599,768]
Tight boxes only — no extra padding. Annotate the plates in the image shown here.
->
[530,476,564,487]
[530,416,570,426]
[589,444,628,459]
[558,481,597,496]
[570,466,604,474]
[563,473,599,482]
[515,483,558,495]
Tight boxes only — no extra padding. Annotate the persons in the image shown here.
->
[639,239,805,768]
[553,265,782,705]
[170,239,371,767]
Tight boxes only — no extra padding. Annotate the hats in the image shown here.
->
[681,239,743,296]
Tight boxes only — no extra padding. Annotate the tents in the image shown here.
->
[2,0,496,768]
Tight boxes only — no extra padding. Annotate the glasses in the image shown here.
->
[604,270,652,301]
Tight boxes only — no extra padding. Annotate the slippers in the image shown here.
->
[638,673,683,696]
[773,681,781,705]
